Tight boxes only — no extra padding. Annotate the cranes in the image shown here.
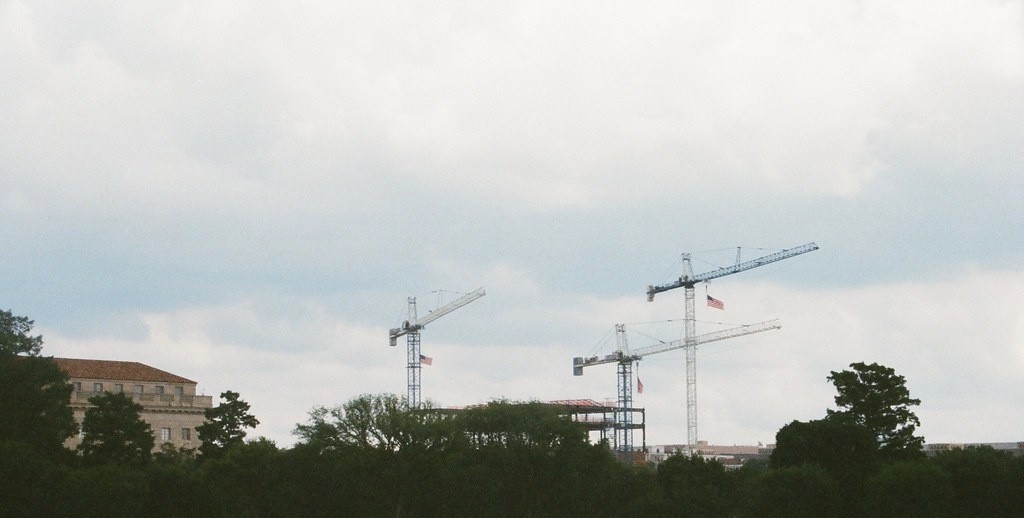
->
[573,317,784,451]
[646,242,821,450]
[389,284,488,412]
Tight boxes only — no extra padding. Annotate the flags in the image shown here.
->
[420,354,432,365]
[707,296,724,310]
[637,379,644,393]
